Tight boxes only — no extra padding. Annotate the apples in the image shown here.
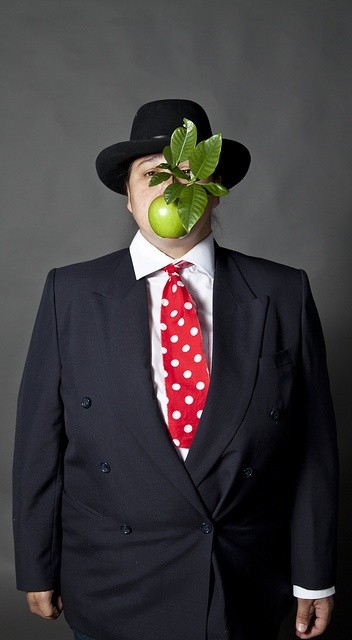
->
[148,194,187,239]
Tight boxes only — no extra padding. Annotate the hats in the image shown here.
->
[96,99,250,195]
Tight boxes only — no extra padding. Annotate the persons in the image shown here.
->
[12,99,340,640]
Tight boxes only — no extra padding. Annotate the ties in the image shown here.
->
[159,261,210,449]
